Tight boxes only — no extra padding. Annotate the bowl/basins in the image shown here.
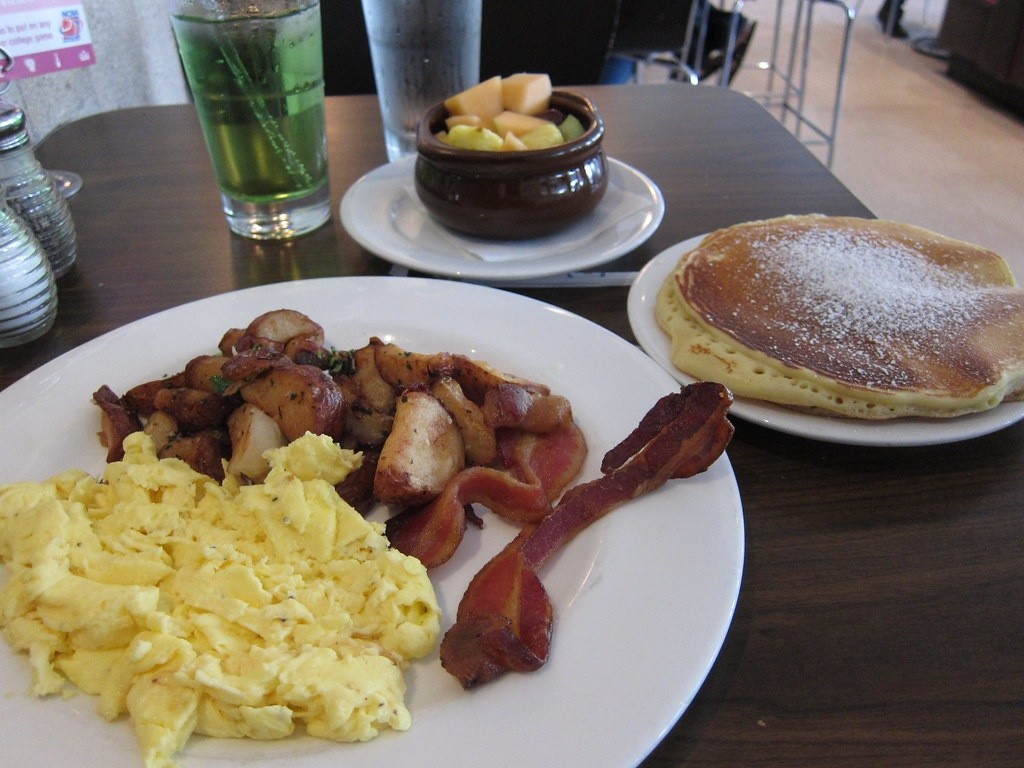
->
[414,90,609,242]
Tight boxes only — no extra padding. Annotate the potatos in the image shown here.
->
[91,307,554,514]
[444,115,584,150]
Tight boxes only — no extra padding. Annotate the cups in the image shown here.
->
[361,0,484,164]
[169,0,332,240]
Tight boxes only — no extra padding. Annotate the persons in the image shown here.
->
[878,0,909,39]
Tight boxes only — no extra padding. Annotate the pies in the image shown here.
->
[654,212,1024,422]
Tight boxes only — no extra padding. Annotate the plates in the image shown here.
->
[0,276,745,768]
[337,151,664,281]
[626,232,1023,445]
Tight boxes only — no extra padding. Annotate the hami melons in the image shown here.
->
[434,72,553,151]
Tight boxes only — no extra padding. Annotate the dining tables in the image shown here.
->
[0,80,1024,768]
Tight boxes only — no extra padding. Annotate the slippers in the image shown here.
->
[670,54,725,84]
[729,19,759,84]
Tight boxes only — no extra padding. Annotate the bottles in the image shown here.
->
[0,102,78,350]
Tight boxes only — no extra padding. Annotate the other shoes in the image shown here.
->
[878,13,908,40]
